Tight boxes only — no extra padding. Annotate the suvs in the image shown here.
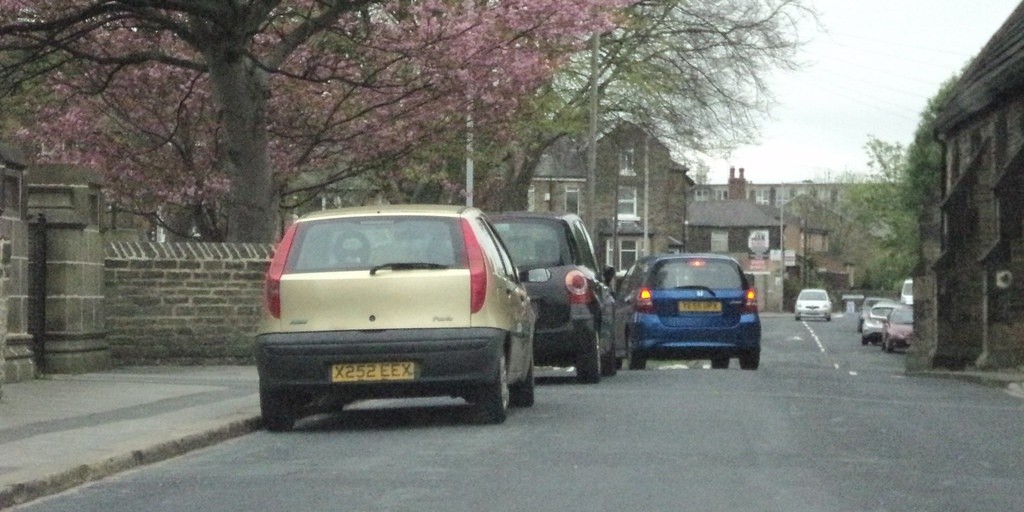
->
[862,301,896,344]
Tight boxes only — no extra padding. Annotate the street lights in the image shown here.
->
[780,192,806,314]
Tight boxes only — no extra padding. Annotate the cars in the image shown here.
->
[879,306,915,353]
[482,212,620,382]
[254,203,536,432]
[794,288,833,323]
[858,296,894,330]
[614,256,762,371]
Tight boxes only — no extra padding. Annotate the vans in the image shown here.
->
[901,279,915,306]
